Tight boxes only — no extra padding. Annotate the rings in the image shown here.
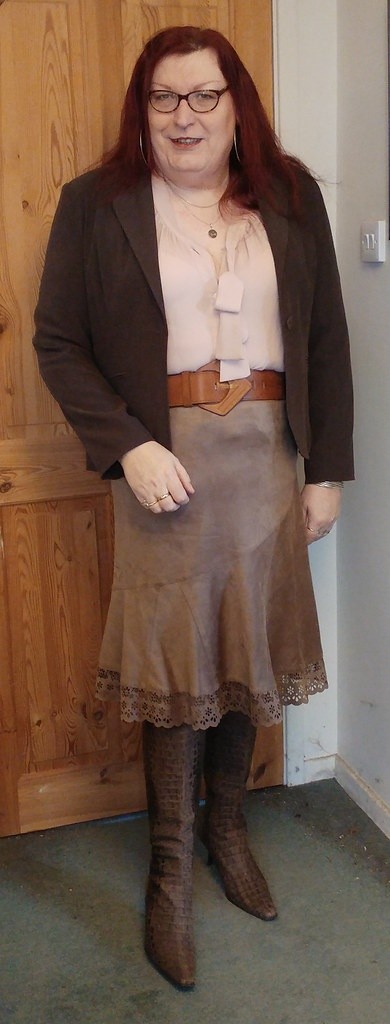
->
[147,501,159,509]
[308,526,314,532]
[157,493,169,499]
[320,532,328,535]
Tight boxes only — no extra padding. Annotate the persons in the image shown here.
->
[33,27,357,988]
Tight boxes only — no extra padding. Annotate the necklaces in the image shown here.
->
[169,184,222,239]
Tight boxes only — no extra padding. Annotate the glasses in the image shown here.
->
[147,85,230,113]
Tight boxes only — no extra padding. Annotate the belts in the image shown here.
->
[166,358,286,416]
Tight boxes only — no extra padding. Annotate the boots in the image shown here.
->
[144,718,201,990]
[202,709,278,922]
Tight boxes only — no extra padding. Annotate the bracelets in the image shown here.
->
[315,478,345,493]
